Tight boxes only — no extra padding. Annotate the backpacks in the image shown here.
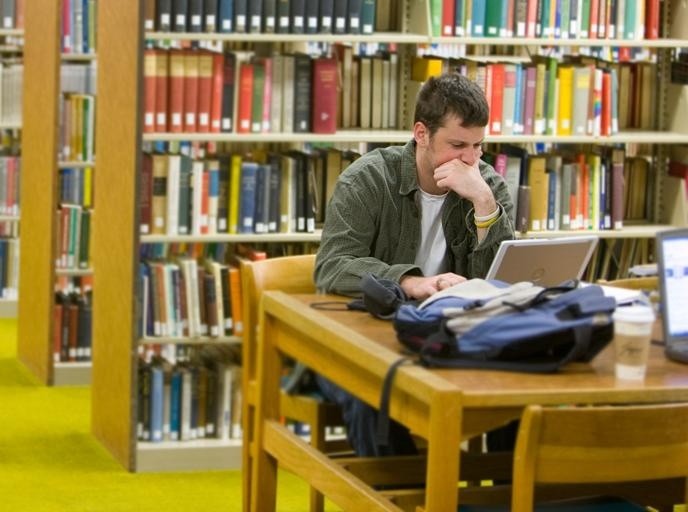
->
[393,279,615,369]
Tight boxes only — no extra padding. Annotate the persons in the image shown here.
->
[285,68,521,493]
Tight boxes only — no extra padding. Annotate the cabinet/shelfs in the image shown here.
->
[90,0,688,475]
[15,0,100,388]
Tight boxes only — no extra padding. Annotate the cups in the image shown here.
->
[612,306,654,382]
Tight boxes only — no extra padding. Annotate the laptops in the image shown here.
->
[485,235,601,292]
[657,227,688,365]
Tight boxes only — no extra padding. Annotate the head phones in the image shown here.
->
[357,271,405,321]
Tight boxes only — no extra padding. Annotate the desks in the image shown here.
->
[248,289,688,512]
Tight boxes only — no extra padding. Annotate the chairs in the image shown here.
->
[239,255,356,512]
[512,403,688,512]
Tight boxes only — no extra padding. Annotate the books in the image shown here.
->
[1,1,22,307]
[54,0,100,368]
[136,0,400,442]
[411,1,688,282]
